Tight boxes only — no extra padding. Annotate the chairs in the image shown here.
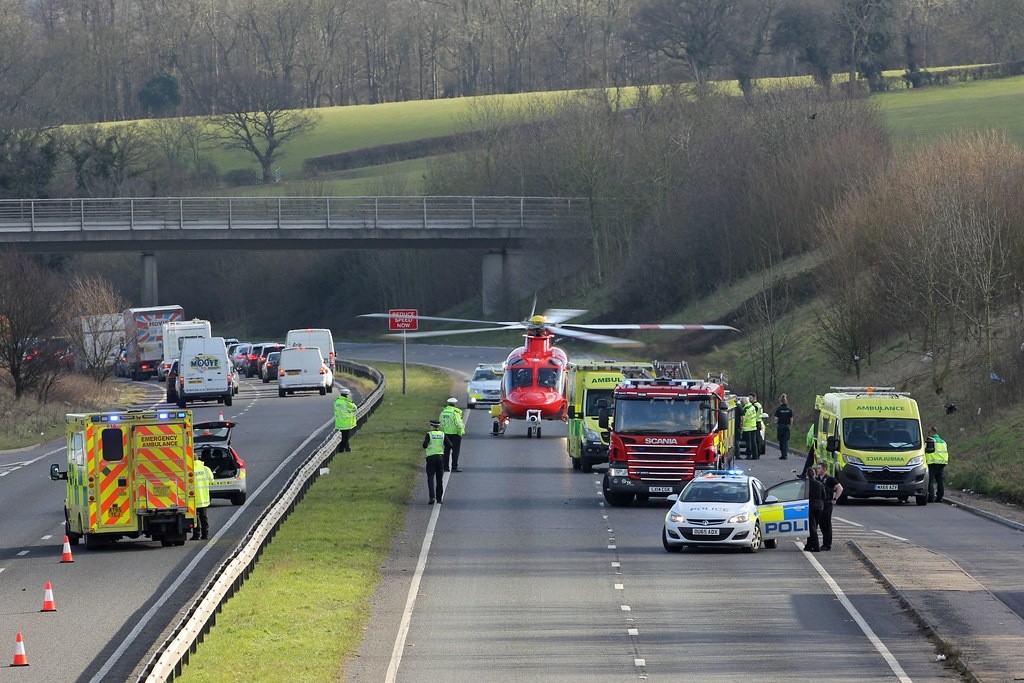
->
[846,421,869,445]
[891,421,912,442]
[699,488,716,501]
[732,491,747,500]
[480,372,488,380]
[200,449,213,467]
[873,420,893,441]
[210,449,227,468]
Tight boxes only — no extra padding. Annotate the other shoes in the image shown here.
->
[437,501,442,504]
[452,468,462,472]
[796,474,806,480]
[444,469,450,472]
[804,546,812,551]
[926,499,933,503]
[779,456,788,460]
[934,499,942,503]
[810,549,820,552]
[428,499,435,505]
[201,536,208,540]
[190,537,199,540]
[820,545,832,551]
[735,455,760,460]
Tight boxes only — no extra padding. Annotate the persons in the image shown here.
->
[189,453,214,540]
[800,463,844,552]
[734,401,745,461]
[749,393,762,458]
[439,398,463,472]
[926,427,948,503]
[796,424,815,480]
[334,389,357,452]
[774,394,793,460]
[422,420,453,505]
[740,398,758,460]
[544,372,556,386]
[518,370,528,385]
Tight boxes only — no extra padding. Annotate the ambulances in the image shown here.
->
[49,407,198,551]
[812,386,931,505]
[565,359,658,473]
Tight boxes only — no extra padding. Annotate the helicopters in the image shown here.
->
[354,292,743,439]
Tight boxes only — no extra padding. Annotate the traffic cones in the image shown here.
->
[59,535,74,563]
[201,417,212,436]
[40,581,57,612]
[218,408,224,421]
[9,632,30,666]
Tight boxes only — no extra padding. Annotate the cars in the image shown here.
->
[112,348,128,377]
[662,471,810,553]
[156,361,167,382]
[463,362,505,408]
[20,338,75,373]
[224,338,285,383]
[193,420,247,506]
[165,359,179,403]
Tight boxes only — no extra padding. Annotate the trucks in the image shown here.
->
[596,359,737,507]
[122,304,186,381]
[69,312,127,375]
[162,317,212,380]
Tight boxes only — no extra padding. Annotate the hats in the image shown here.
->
[340,389,350,395]
[428,419,441,426]
[447,397,458,404]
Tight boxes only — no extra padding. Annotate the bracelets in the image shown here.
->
[834,498,836,500]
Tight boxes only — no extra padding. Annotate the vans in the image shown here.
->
[176,336,243,409]
[278,328,338,397]
